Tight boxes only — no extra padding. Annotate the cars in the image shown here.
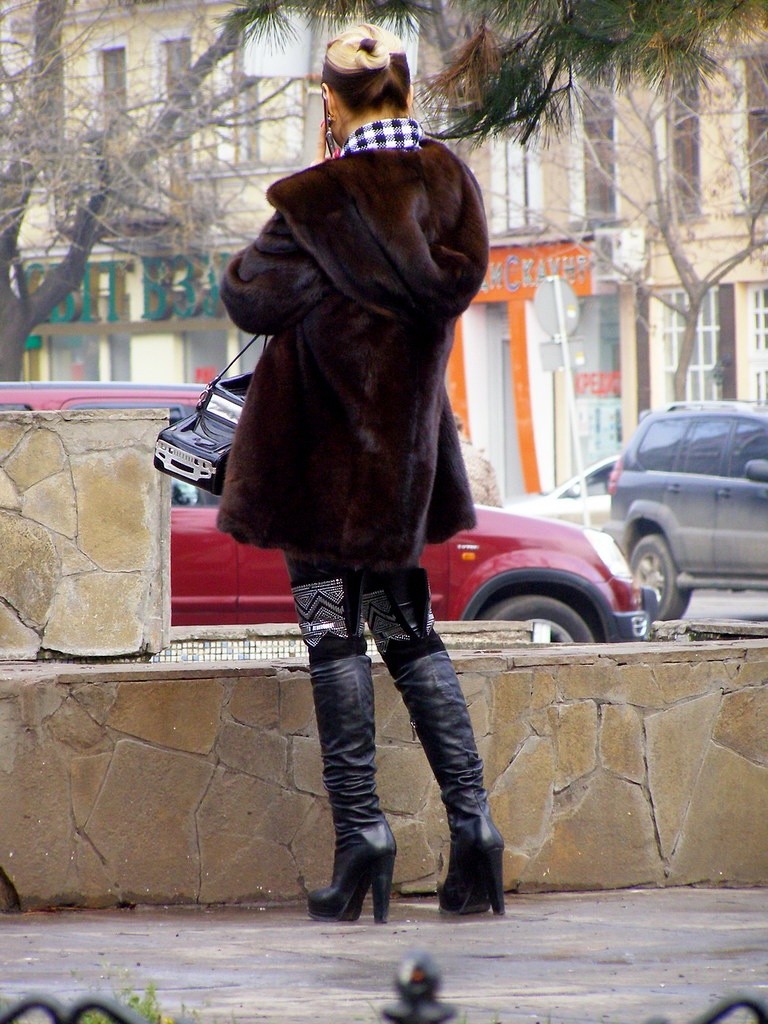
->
[0,381,660,649]
[497,452,620,534]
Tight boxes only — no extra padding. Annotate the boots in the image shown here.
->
[306,656,397,924]
[394,650,505,916]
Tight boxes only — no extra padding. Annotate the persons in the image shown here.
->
[452,413,501,508]
[220,23,504,924]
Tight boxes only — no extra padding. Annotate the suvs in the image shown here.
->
[606,400,768,622]
